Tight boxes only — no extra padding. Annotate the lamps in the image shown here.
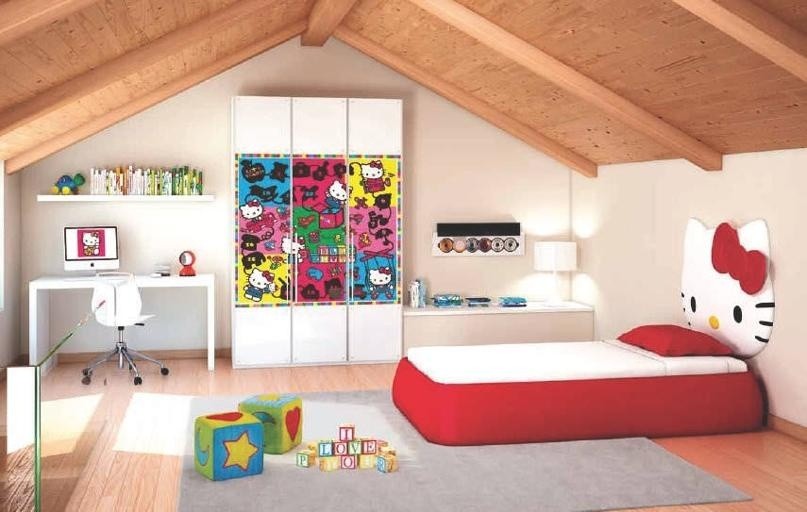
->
[530,240,581,311]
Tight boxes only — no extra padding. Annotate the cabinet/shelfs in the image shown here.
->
[230,94,405,371]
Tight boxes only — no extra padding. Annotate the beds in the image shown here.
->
[391,341,767,448]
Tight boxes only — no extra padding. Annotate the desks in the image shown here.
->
[26,271,221,382]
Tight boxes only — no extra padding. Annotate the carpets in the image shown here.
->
[174,389,754,511]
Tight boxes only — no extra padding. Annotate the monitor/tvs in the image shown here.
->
[63,225,120,280]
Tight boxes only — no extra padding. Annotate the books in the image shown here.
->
[90,163,202,194]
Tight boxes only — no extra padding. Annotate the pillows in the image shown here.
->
[613,323,740,357]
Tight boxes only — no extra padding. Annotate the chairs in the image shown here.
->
[78,282,173,386]
